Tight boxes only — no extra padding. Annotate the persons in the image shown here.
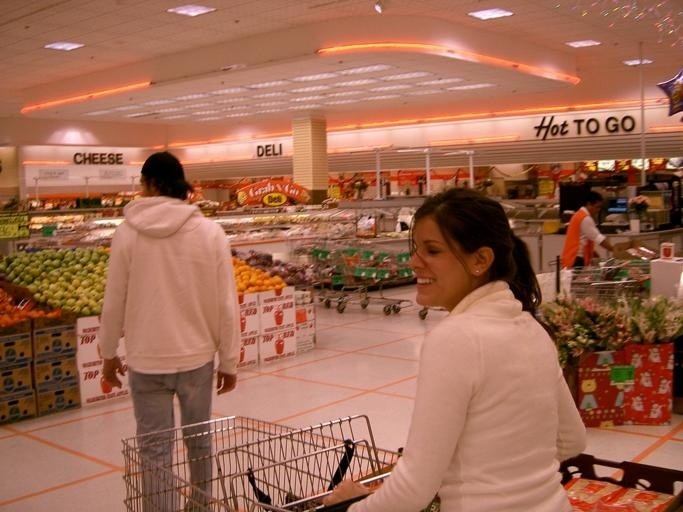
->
[322,187,588,512]
[560,191,615,278]
[98,151,240,512]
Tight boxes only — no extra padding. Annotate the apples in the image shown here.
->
[0,247,111,316]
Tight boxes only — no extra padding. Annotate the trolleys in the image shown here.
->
[301,237,453,319]
[120,414,404,512]
[569,257,650,301]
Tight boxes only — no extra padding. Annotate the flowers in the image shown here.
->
[536,293,681,360]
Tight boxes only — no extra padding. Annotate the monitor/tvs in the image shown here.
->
[606,196,628,214]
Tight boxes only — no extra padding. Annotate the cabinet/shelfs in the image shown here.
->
[74,210,356,265]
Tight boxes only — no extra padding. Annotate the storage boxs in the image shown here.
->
[4,286,128,426]
[239,286,317,367]
[639,189,674,225]
